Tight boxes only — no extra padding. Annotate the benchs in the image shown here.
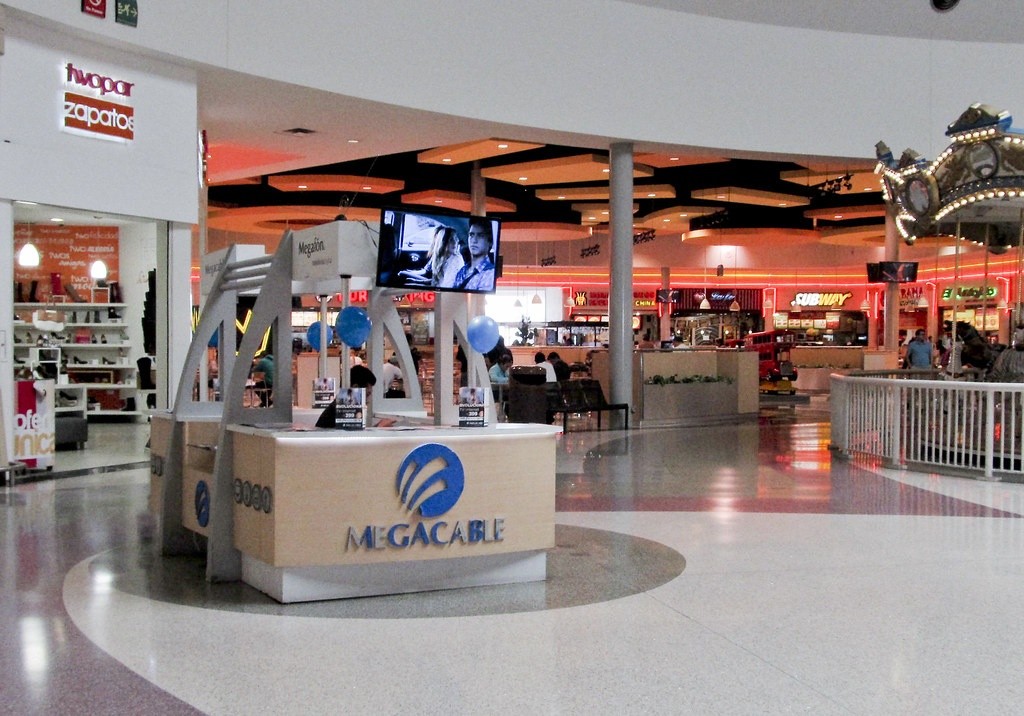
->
[544,380,629,430]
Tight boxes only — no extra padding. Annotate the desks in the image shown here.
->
[491,383,508,423]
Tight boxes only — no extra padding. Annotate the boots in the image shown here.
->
[15,268,158,408]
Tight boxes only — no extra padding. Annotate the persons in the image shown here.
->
[399,224,467,288]
[252,349,274,408]
[490,353,516,418]
[547,350,571,381]
[452,216,496,291]
[535,352,556,382]
[348,334,421,397]
[455,341,468,388]
[488,336,513,366]
[316,364,393,429]
[907,328,931,369]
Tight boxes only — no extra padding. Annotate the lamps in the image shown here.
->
[19,219,39,266]
[268,138,886,235]
[564,295,575,309]
[514,300,521,309]
[533,292,541,303]
[92,216,107,278]
[729,300,739,311]
[700,297,711,309]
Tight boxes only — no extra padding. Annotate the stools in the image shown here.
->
[55,417,87,449]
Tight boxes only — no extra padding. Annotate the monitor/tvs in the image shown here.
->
[375,206,502,295]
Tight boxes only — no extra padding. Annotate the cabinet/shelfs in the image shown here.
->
[143,402,562,602]
